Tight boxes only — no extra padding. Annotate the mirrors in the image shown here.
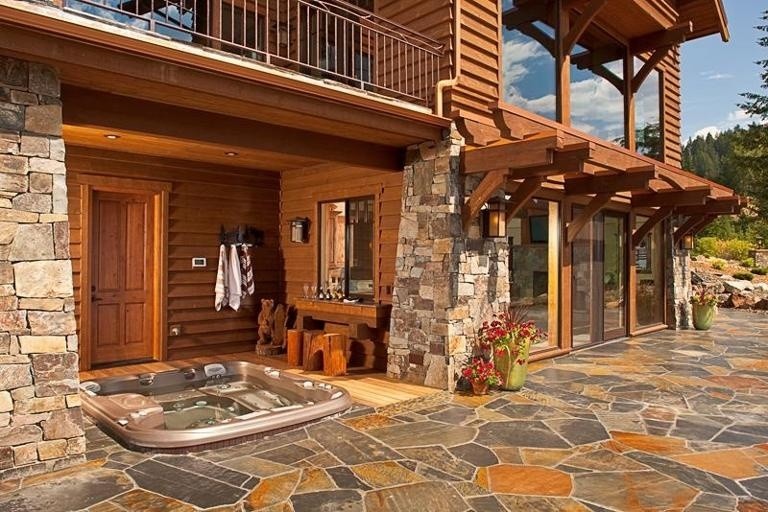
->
[316,194,376,304]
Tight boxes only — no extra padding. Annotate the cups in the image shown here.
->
[302,285,318,299]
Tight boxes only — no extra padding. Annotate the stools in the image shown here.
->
[302,328,348,376]
[287,328,309,367]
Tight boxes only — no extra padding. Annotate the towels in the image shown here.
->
[215,244,228,311]
[226,243,242,313]
[240,243,255,300]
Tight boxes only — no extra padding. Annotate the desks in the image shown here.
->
[294,297,392,369]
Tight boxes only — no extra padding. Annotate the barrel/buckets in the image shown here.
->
[492,338,530,393]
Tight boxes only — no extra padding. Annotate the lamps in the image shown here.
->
[680,233,694,251]
[289,216,311,243]
[273,21,292,48]
[479,196,507,238]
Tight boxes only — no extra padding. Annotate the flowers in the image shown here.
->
[473,304,548,366]
[463,339,503,388]
[687,282,720,311]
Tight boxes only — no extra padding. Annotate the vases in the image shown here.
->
[494,353,528,392]
[470,378,488,395]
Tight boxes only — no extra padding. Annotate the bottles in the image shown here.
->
[319,287,324,300]
[325,288,331,300]
